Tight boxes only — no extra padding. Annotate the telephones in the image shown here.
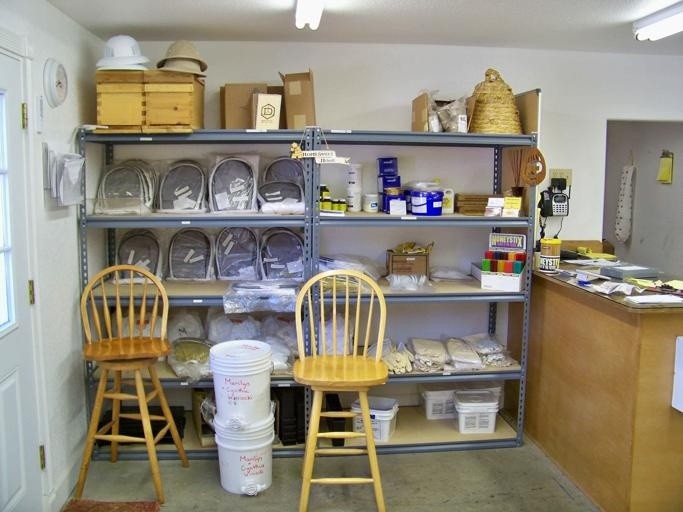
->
[540,191,568,217]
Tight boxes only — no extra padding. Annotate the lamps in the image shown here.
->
[293,0,326,31]
[632,1,682,43]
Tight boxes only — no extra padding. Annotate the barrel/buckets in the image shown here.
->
[410,188,456,215]
[215,426,275,495]
[539,237,562,274]
[209,340,274,431]
[352,396,399,442]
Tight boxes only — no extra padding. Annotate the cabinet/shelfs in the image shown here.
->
[74,130,312,461]
[305,129,535,457]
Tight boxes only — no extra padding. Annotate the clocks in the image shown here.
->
[43,57,68,107]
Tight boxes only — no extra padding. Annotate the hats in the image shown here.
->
[96,35,149,71]
[156,40,208,78]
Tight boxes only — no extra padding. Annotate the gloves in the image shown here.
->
[381,332,511,375]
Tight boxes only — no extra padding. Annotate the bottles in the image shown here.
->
[540,237,560,275]
[319,183,345,216]
[347,164,377,215]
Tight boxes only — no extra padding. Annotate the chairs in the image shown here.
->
[287,270,393,512]
[74,265,189,505]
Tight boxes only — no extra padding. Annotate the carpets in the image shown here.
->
[62,497,163,511]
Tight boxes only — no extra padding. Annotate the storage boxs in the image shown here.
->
[218,77,267,129]
[387,250,429,284]
[349,381,501,441]
[277,66,316,129]
